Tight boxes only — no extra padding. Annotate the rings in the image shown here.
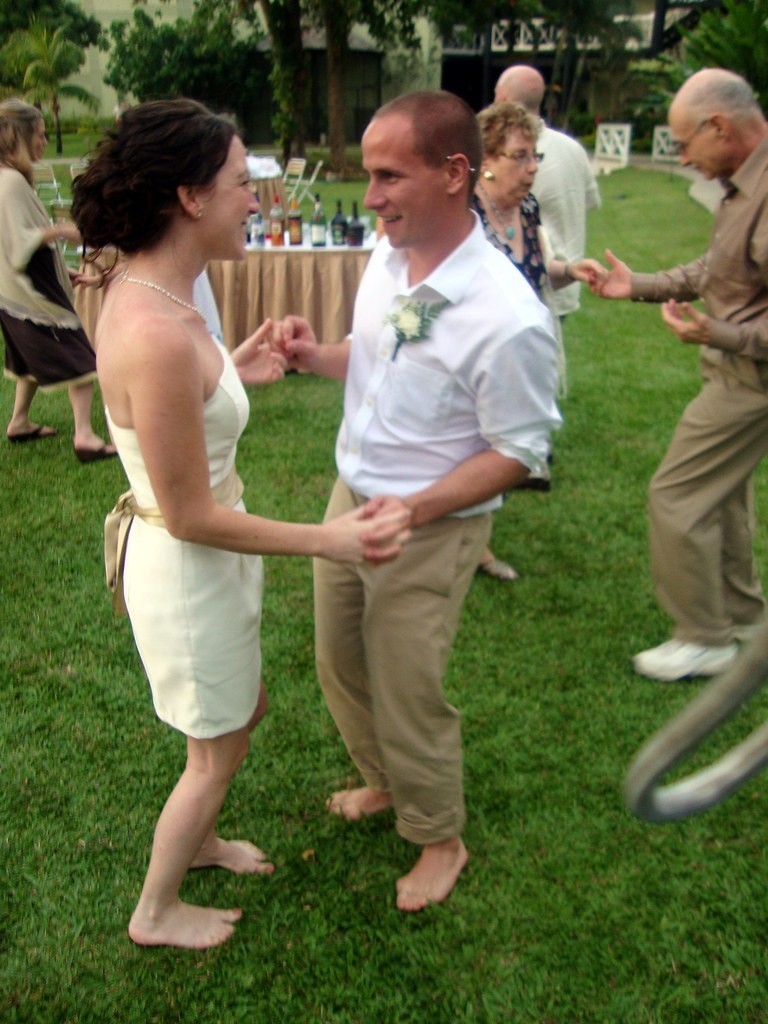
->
[394,538,404,547]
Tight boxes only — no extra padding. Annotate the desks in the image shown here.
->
[245,155,289,229]
[73,223,376,374]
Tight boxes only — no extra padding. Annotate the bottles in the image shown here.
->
[269,195,285,247]
[288,200,302,245]
[247,184,264,243]
[311,194,328,245]
[241,213,264,245]
[333,200,365,248]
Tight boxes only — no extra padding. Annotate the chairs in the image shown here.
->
[31,162,92,268]
[282,158,323,206]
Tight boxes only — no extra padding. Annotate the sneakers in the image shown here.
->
[731,602,768,641]
[633,637,739,681]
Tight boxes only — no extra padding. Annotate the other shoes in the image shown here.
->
[475,558,521,580]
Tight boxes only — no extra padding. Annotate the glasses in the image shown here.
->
[499,151,544,164]
[671,120,709,150]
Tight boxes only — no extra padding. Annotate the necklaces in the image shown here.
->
[121,272,207,322]
[475,179,515,241]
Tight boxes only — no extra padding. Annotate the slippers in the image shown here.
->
[8,423,59,444]
[74,439,118,464]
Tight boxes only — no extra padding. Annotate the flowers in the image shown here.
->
[389,297,449,361]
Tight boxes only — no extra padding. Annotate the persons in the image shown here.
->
[270,91,564,913]
[467,68,768,682]
[70,102,411,949]
[0,100,117,463]
